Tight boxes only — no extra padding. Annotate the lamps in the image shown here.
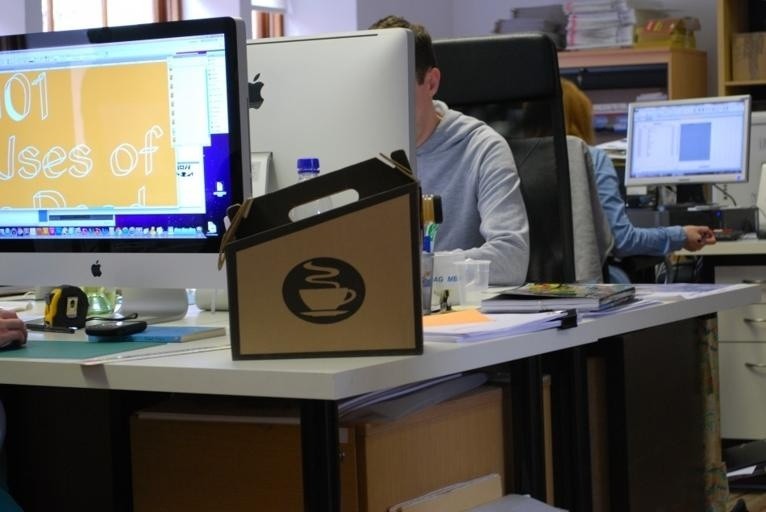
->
[426,33,570,298]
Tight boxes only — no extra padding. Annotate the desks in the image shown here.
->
[0,276,763,512]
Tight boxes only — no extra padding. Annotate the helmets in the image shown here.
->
[301,310,349,317]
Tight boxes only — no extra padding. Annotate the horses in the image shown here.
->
[84,322,143,337]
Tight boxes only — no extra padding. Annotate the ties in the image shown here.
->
[196,27,418,303]
[624,94,753,205]
[428,32,577,285]
[0,16,253,325]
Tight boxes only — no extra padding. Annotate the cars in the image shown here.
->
[453,260,491,310]
[432,253,480,308]
[299,288,356,310]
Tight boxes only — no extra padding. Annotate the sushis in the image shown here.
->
[84,319,148,336]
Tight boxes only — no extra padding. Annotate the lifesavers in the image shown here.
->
[622,203,756,240]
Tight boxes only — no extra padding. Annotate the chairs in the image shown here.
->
[426,33,570,298]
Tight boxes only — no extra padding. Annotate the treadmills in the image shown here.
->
[45,286,90,327]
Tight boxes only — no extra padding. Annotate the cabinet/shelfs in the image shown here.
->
[291,157,334,221]
[707,263,765,441]
[518,0,766,206]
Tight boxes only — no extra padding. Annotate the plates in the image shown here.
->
[707,263,765,441]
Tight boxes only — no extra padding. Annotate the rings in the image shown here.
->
[26,324,74,333]
[420,221,440,256]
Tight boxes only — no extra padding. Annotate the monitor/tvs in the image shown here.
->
[422,304,578,347]
[481,278,636,315]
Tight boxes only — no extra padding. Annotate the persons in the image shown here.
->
[0,305,28,354]
[370,12,533,287]
[561,76,718,285]
